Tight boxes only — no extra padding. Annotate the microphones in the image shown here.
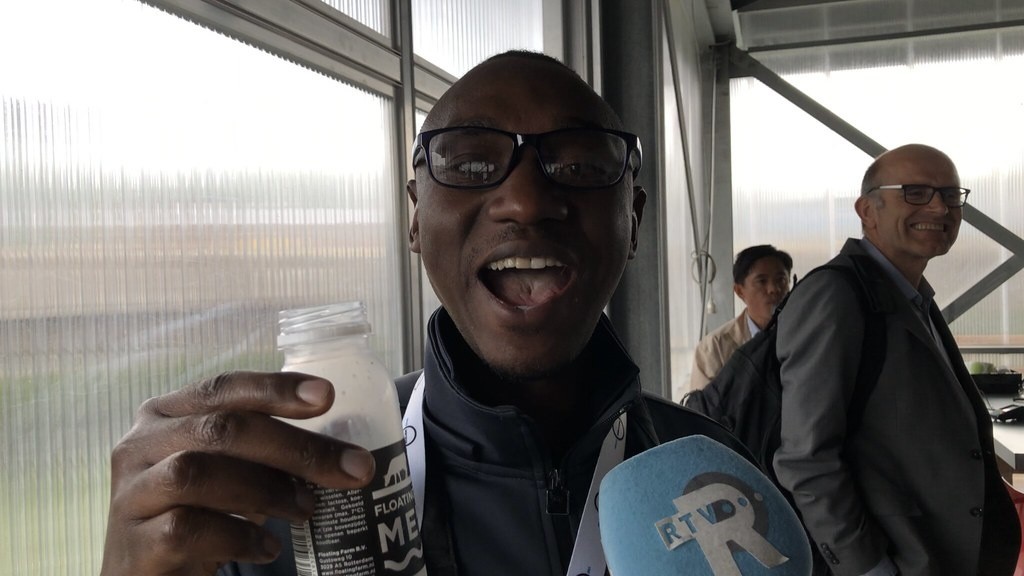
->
[598,434,814,576]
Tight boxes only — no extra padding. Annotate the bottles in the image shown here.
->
[277,301,428,576]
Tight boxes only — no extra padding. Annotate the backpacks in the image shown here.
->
[684,262,885,491]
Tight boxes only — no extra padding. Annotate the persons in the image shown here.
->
[99,50,837,576]
[691,244,794,391]
[765,143,1021,575]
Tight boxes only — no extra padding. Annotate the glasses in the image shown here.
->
[868,182,971,209]
[412,123,644,192]
